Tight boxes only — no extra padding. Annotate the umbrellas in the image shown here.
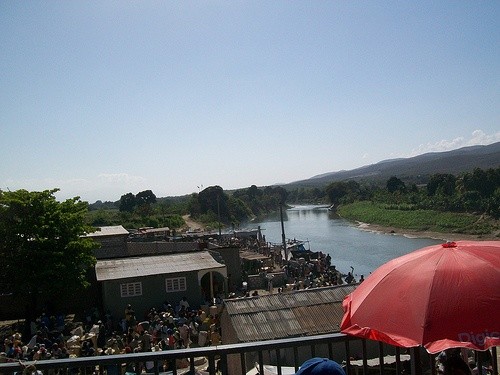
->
[339,240,500,375]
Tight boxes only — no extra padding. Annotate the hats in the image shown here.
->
[295,357,346,375]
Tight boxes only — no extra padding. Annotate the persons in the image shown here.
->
[434,346,475,375]
[0,296,231,375]
[170,225,366,299]
[468,347,495,375]
[296,358,346,374]
